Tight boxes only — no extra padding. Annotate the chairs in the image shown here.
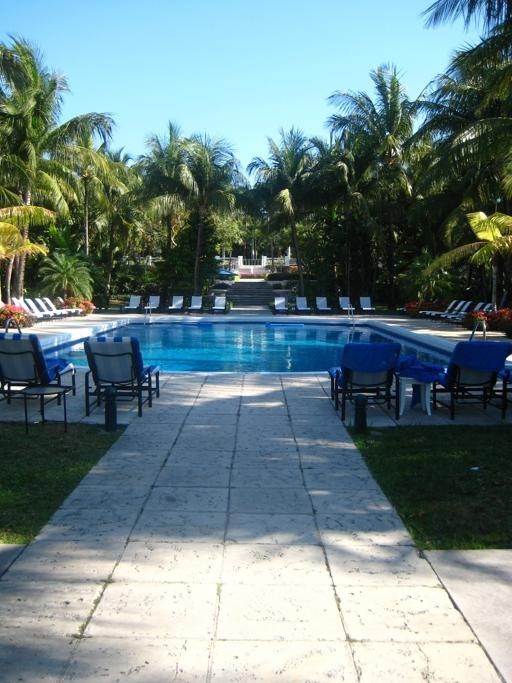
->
[168,295,183,313]
[211,297,226,314]
[339,296,355,315]
[295,297,311,314]
[12,297,83,319]
[274,296,289,315]
[360,297,376,315]
[84,336,160,416]
[498,367,512,419]
[124,295,142,313]
[188,296,202,313]
[144,295,160,314]
[328,343,401,422]
[418,300,494,328]
[0,332,76,405]
[315,297,332,315]
[430,341,512,420]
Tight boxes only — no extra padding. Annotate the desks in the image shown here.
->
[21,385,73,433]
[395,369,432,415]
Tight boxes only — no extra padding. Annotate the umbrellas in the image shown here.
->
[207,268,239,277]
